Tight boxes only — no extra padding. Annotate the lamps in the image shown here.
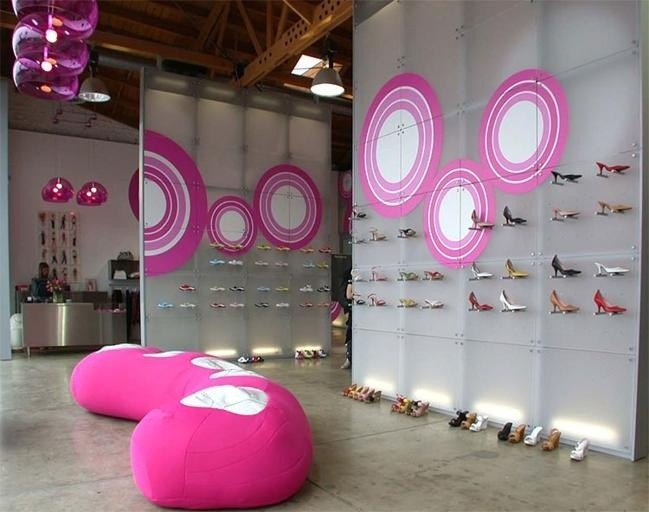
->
[41,110,108,207]
[309,49,346,98]
[11,0,112,104]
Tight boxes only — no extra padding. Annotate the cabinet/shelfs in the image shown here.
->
[347,169,626,318]
[157,246,331,311]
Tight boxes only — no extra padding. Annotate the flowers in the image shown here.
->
[45,276,67,293]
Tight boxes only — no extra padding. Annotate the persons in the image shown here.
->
[31,263,53,302]
[341,278,353,369]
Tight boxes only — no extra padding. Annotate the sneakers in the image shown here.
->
[295,349,328,359]
[157,243,330,308]
[237,356,263,363]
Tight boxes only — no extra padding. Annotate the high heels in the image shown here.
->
[447,408,488,432]
[352,293,365,305]
[599,200,629,214]
[340,385,382,403]
[398,299,416,307]
[369,229,385,241]
[423,299,444,308]
[594,262,629,275]
[350,233,364,243]
[472,209,495,229]
[371,268,387,281]
[551,255,581,277]
[551,170,582,182]
[391,392,431,418]
[352,207,366,218]
[505,260,527,277]
[500,290,526,311]
[499,423,590,460]
[398,227,416,236]
[351,269,363,281]
[398,271,417,280]
[554,207,580,218]
[469,292,492,309]
[503,206,527,225]
[594,289,625,313]
[471,262,492,278]
[369,293,385,306]
[596,161,629,174]
[423,270,443,279]
[550,290,578,312]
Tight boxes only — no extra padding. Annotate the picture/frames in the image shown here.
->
[85,279,97,292]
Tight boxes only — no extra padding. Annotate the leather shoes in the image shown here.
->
[341,358,350,369]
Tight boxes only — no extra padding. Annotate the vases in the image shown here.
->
[53,292,63,303]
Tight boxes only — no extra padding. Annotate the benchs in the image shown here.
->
[69,342,313,511]
